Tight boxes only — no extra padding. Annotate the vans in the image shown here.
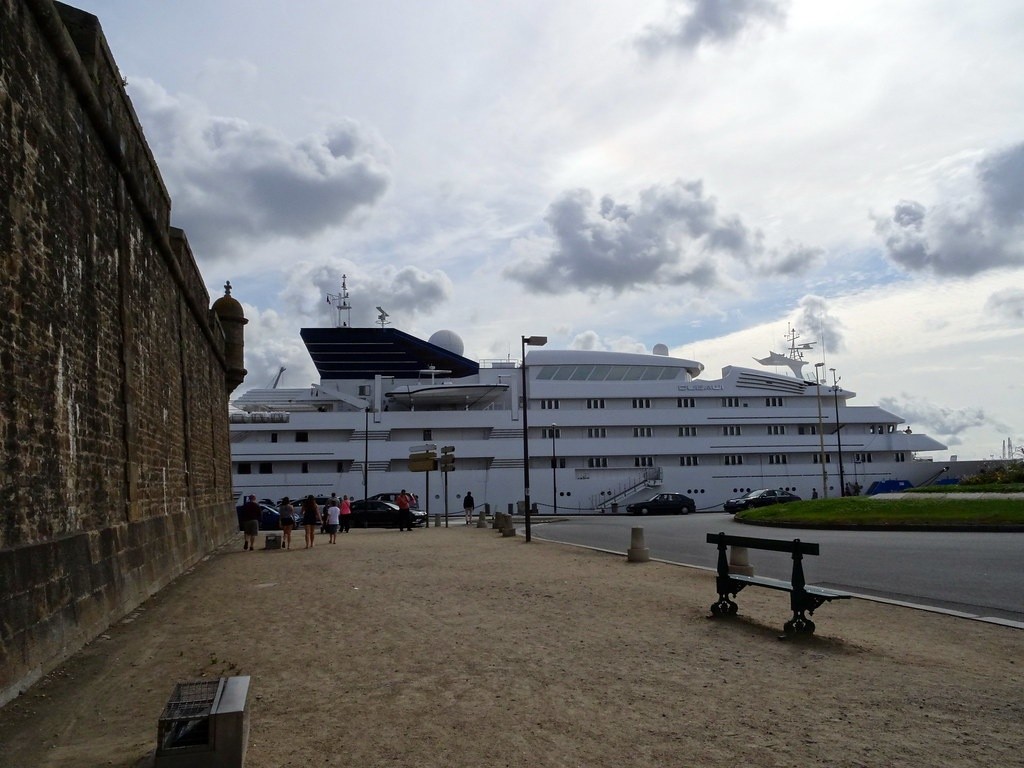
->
[278,498,330,520]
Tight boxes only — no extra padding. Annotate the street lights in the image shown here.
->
[365,405,370,499]
[814,363,828,497]
[829,367,844,496]
[521,334,548,545]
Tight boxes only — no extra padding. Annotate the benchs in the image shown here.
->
[705,533,852,641]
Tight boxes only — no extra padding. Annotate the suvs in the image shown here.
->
[369,492,417,510]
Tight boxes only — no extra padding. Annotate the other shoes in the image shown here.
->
[244,541,248,550]
[250,546,254,551]
[400,529,403,532]
[407,529,413,531]
[282,541,286,548]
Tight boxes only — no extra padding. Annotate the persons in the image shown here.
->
[326,493,339,534]
[279,497,296,549]
[300,495,323,549]
[339,495,351,533]
[811,488,819,500]
[411,493,419,510]
[463,491,474,525]
[903,426,912,434]
[848,482,860,496]
[242,494,262,551]
[325,501,340,544]
[396,489,413,531]
[349,496,355,502]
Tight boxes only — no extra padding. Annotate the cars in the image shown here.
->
[724,488,802,514]
[349,500,427,527]
[626,492,696,516]
[237,504,301,530]
